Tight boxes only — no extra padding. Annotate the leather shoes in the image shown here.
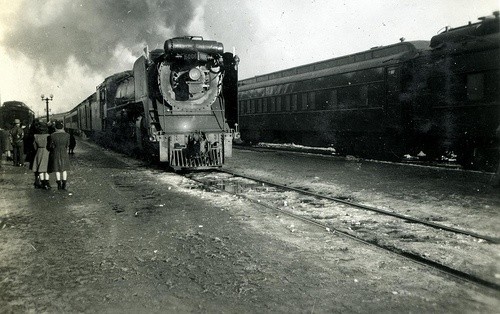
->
[19,164,26,167]
[13,163,20,166]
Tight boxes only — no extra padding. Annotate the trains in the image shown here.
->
[237,11,500,172]
[0,101,34,128]
[64,36,241,172]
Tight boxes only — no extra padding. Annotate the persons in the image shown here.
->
[25,119,42,169]
[49,120,70,191]
[33,123,51,190]
[69,131,76,154]
[9,118,25,167]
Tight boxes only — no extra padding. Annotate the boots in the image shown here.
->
[45,180,51,190]
[40,180,44,189]
[62,180,67,191]
[56,181,61,190]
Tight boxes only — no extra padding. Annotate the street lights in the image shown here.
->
[41,94,53,122]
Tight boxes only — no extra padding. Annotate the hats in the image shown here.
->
[15,119,21,123]
